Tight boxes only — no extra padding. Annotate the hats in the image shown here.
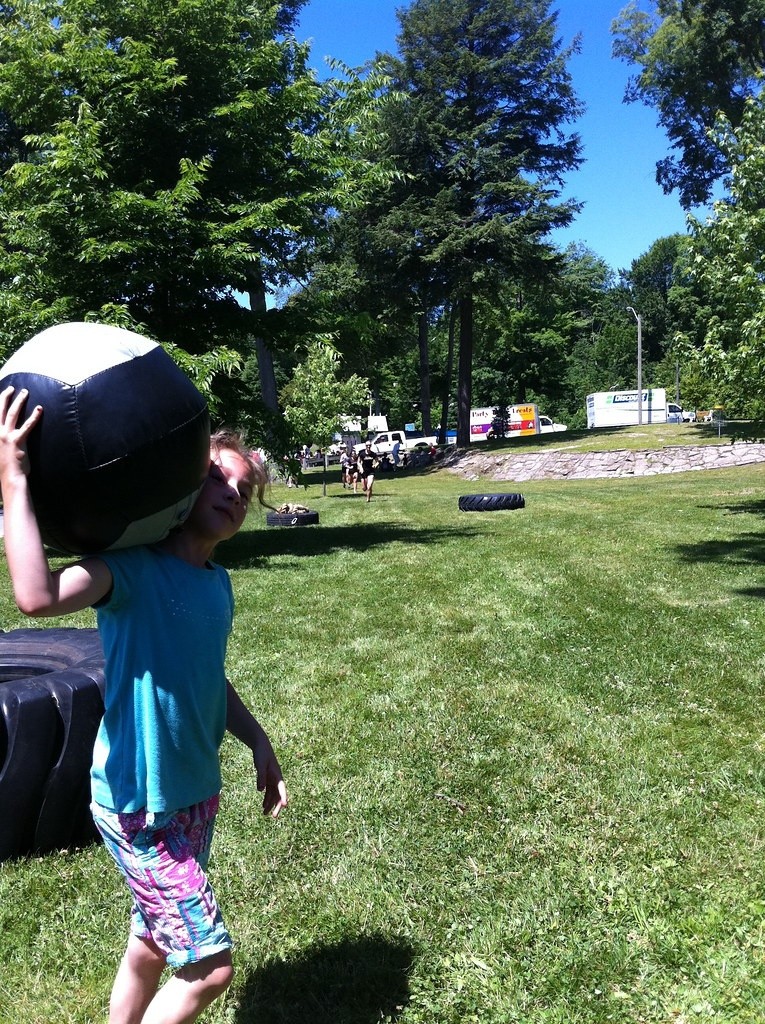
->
[365,441,372,446]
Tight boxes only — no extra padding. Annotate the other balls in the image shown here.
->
[0,320,212,557]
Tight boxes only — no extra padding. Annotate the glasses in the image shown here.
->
[366,445,371,446]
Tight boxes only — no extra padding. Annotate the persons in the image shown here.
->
[340,447,349,488]
[344,451,358,494]
[0,384,289,1024]
[392,440,404,472]
[357,441,379,502]
[286,442,437,470]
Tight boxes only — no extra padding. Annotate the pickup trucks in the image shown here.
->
[352,430,439,457]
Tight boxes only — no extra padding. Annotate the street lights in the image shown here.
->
[626,307,641,425]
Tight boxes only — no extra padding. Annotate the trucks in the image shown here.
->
[469,403,567,442]
[586,388,696,428]
